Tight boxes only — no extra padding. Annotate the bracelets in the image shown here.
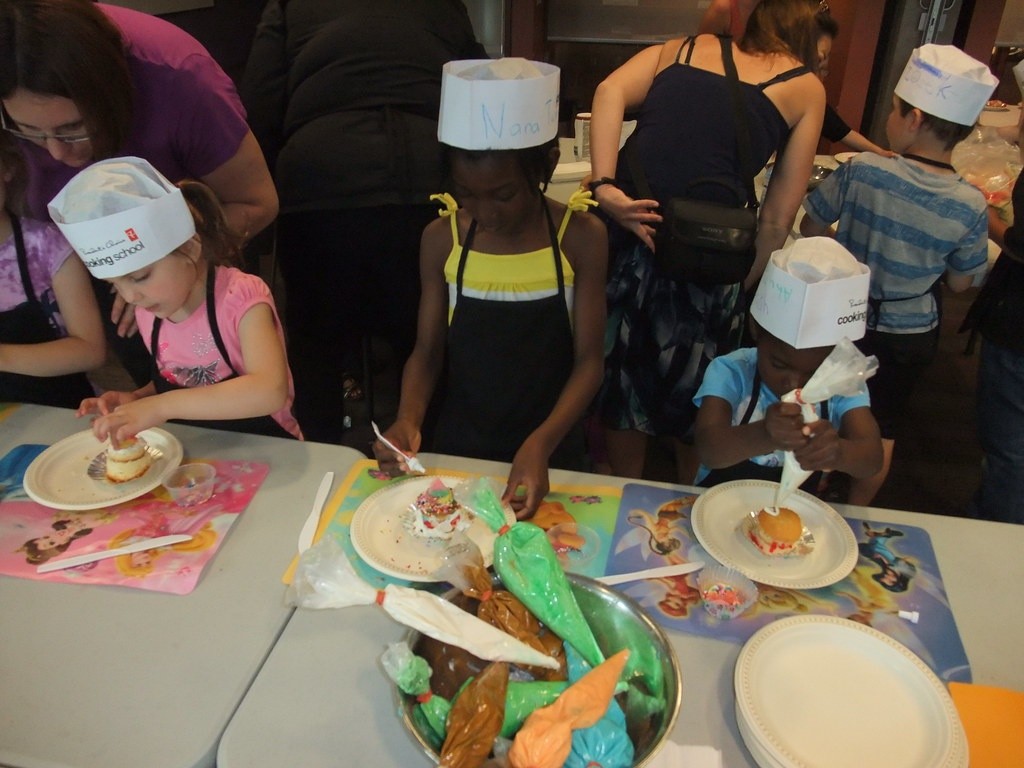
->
[588,177,624,191]
[236,208,252,251]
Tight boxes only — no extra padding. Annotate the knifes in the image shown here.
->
[299,472,333,556]
[36,534,192,574]
[593,561,705,586]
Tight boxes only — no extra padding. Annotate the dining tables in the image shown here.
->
[0,403,1024,768]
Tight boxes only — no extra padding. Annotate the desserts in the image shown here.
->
[105,436,153,482]
[751,508,804,557]
[414,478,475,538]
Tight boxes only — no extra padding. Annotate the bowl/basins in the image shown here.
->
[162,462,215,505]
[392,572,681,768]
[546,523,600,570]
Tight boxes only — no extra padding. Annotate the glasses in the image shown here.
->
[0,107,91,142]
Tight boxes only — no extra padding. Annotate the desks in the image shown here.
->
[540,137,1003,267]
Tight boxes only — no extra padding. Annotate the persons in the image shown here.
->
[0,0,307,442]
[799,42,989,506]
[690,236,884,496]
[587,0,897,482]
[242,0,492,447]
[946,110,1024,525]
[341,368,364,401]
[371,56,609,522]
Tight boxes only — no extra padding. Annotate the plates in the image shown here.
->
[690,479,858,590]
[733,614,969,768]
[835,152,861,164]
[765,161,835,192]
[350,476,517,582]
[23,426,183,510]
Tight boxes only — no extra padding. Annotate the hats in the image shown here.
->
[47,156,195,279]
[750,236,871,349]
[894,43,999,126]
[437,57,560,150]
